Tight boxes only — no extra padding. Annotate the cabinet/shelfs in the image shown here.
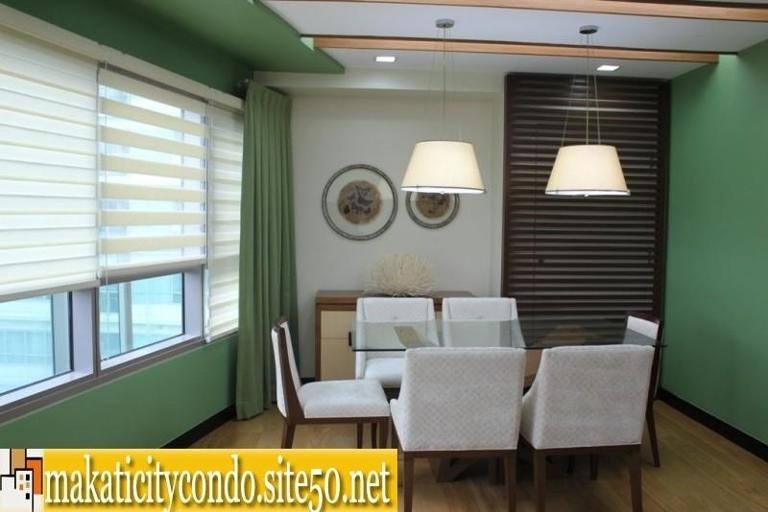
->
[314,290,475,379]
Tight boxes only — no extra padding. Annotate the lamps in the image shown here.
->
[400,17,487,196]
[545,24,632,198]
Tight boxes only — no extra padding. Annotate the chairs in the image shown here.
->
[589,308,668,480]
[354,297,441,389]
[443,296,527,348]
[271,315,392,448]
[386,346,528,512]
[519,343,656,511]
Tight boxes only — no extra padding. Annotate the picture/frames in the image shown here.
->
[405,192,459,229]
[321,163,398,241]
